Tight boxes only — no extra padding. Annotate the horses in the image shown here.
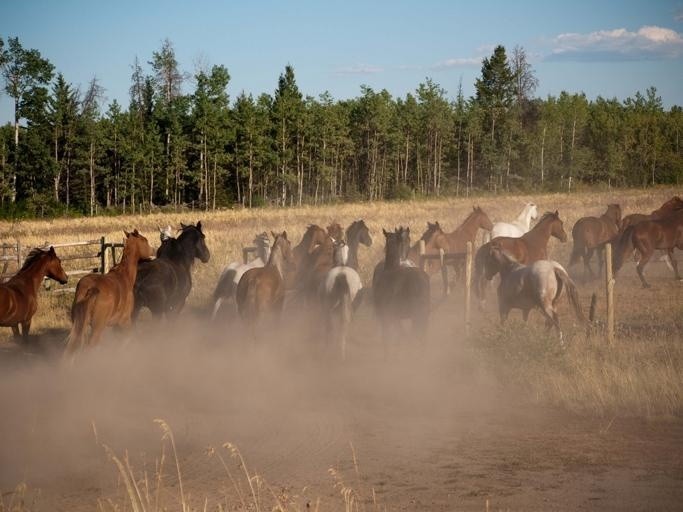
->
[211,219,374,351]
[132,220,211,336]
[58,227,157,365]
[0,244,70,343]
[570,194,682,290]
[367,201,584,356]
